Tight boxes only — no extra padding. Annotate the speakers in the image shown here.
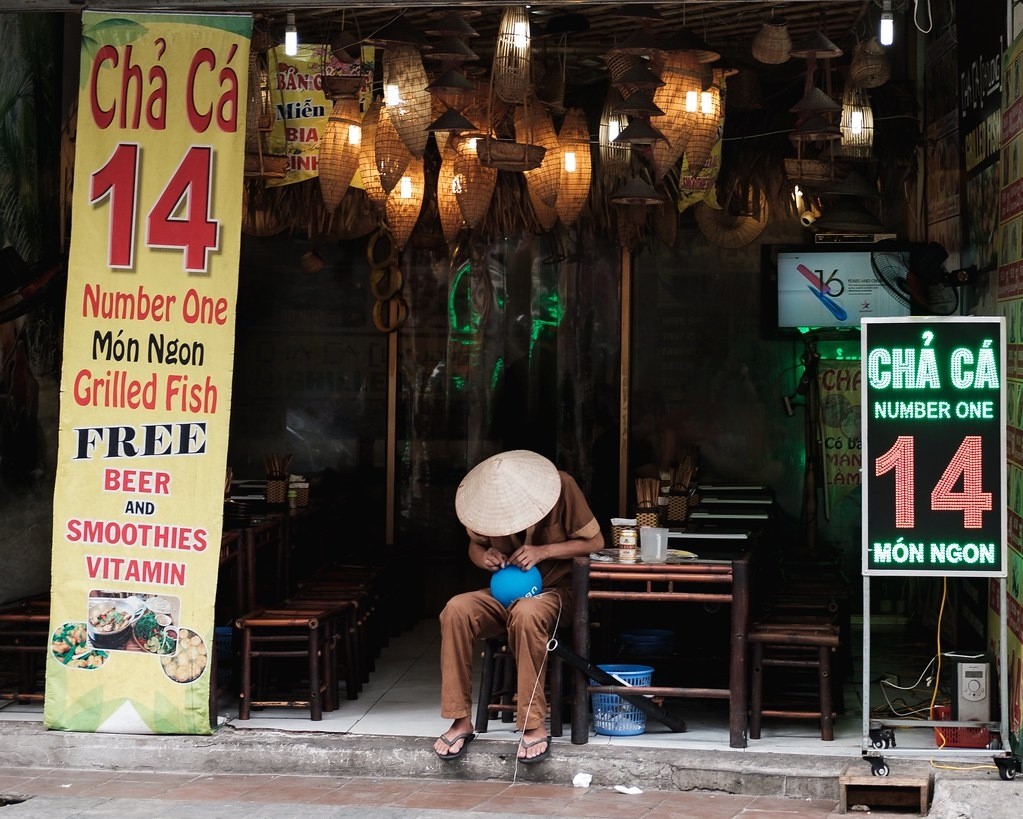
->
[950,653,1000,744]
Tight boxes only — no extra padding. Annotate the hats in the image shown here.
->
[455,449,561,537]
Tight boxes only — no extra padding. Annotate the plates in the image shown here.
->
[132,610,177,656]
[90,599,135,636]
[51,618,112,670]
[154,614,172,627]
[163,625,178,641]
[145,597,172,615]
[159,627,207,686]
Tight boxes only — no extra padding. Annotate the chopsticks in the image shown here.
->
[263,449,295,474]
[635,476,661,508]
[668,456,697,491]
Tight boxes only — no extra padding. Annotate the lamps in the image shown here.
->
[284,1,896,270]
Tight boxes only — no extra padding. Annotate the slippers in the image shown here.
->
[434,733,476,760]
[518,736,552,763]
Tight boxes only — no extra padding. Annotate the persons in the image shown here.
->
[616,405,677,515]
[432,450,605,763]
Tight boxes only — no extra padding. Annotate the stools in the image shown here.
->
[840,765,931,817]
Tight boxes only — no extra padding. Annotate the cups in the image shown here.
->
[640,526,670,564]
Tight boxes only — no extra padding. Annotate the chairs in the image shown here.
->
[1,472,843,748]
